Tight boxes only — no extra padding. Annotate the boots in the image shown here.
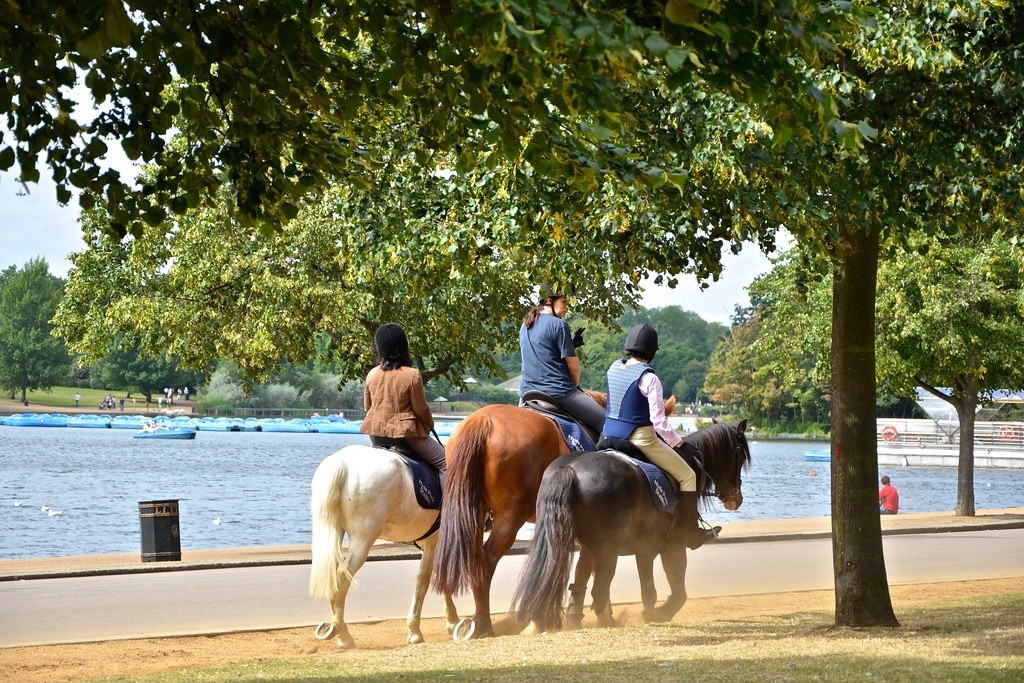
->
[679,491,722,550]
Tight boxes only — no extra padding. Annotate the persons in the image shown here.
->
[158,397,162,409]
[132,398,135,410]
[151,420,156,428]
[598,324,722,550]
[360,323,447,495]
[120,396,124,411]
[166,397,171,409]
[518,280,606,434]
[144,420,149,430]
[164,387,189,400]
[100,394,116,410]
[75,393,80,407]
[879,475,899,514]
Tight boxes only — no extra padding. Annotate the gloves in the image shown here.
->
[678,442,700,462]
[573,327,585,349]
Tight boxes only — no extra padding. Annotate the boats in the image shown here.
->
[429,421,460,437]
[0,413,364,434]
[133,428,196,440]
[804,447,831,462]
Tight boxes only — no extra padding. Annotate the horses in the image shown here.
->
[426,402,614,637]
[305,436,452,652]
[504,419,752,626]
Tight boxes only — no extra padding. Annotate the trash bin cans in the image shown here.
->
[138,499,182,562]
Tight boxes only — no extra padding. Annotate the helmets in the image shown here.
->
[538,281,561,302]
[375,324,408,362]
[625,324,659,355]
